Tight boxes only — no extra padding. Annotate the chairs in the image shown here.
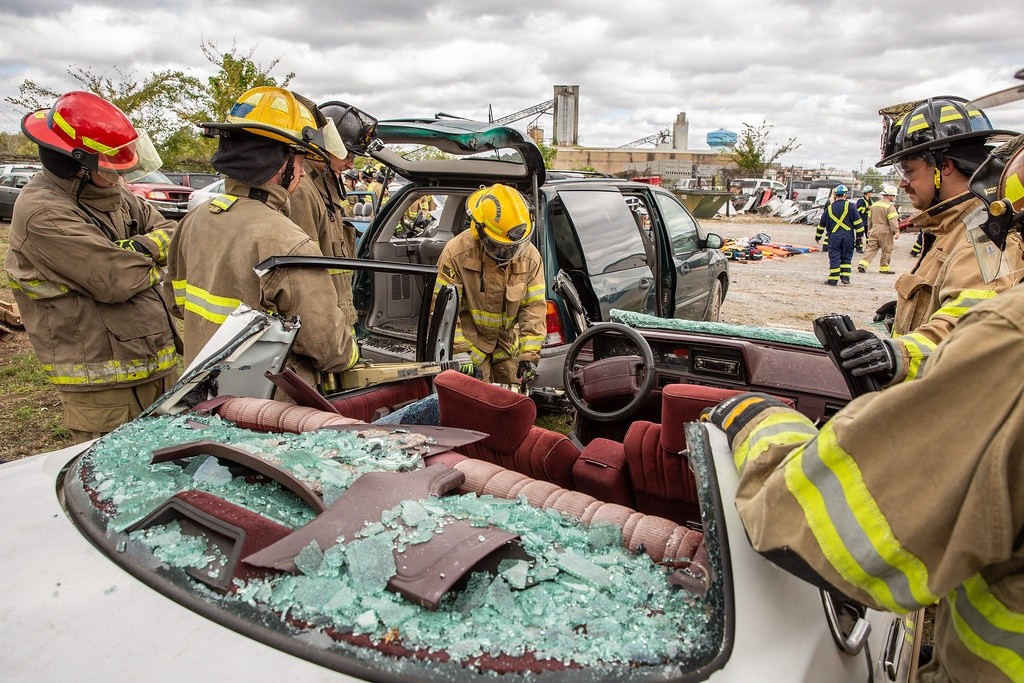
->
[354,202,373,222]
[417,239,446,267]
[433,367,579,483]
[579,390,800,506]
[631,210,655,267]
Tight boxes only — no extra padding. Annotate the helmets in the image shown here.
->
[21,91,142,173]
[834,185,849,195]
[465,183,532,245]
[862,186,873,193]
[318,101,371,158]
[225,85,326,161]
[997,146,1024,228]
[875,96,1023,168]
[879,186,899,196]
[344,164,396,179]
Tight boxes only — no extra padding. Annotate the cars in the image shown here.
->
[1,253,920,683]
[187,179,225,214]
[387,182,446,223]
[785,181,850,201]
[0,164,43,222]
[164,173,222,190]
[122,170,195,218]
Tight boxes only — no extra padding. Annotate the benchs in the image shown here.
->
[225,395,707,579]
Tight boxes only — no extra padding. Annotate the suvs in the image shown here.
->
[349,114,730,419]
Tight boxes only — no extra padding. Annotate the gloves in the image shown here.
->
[113,239,151,256]
[839,330,895,384]
[452,352,474,376]
[894,232,899,239]
[872,301,897,322]
[699,393,776,432]
[867,230,870,237]
[516,361,536,383]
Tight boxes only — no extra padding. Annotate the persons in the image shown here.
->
[815,184,864,286]
[6,91,178,444]
[342,163,437,235]
[857,185,875,253]
[858,186,900,274]
[821,188,837,251]
[908,230,922,257]
[427,183,547,396]
[840,97,1024,388]
[699,135,1024,683]
[163,85,378,403]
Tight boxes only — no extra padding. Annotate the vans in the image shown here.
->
[730,178,788,198]
[671,178,707,191]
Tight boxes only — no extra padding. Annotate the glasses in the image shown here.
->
[893,152,936,183]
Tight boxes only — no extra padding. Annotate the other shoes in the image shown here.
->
[823,247,895,286]
